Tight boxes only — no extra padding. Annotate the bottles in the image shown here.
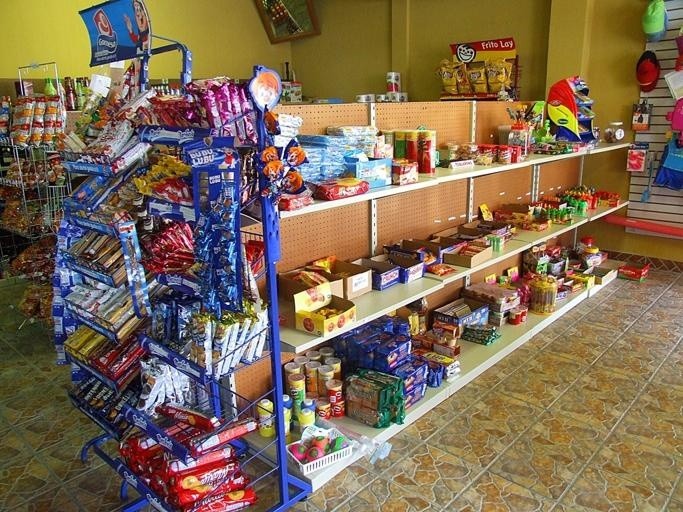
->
[582,246,602,270]
[509,123,529,159]
[152,75,179,96]
[258,395,315,437]
[44,77,88,112]
[604,121,626,143]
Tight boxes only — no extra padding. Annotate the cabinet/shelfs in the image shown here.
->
[0,55,632,512]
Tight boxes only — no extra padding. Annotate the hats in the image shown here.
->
[641,0,669,42]
[636,49,661,92]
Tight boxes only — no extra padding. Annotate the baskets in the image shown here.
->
[285,428,354,474]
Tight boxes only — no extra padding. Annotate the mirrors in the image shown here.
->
[254,0,321,45]
